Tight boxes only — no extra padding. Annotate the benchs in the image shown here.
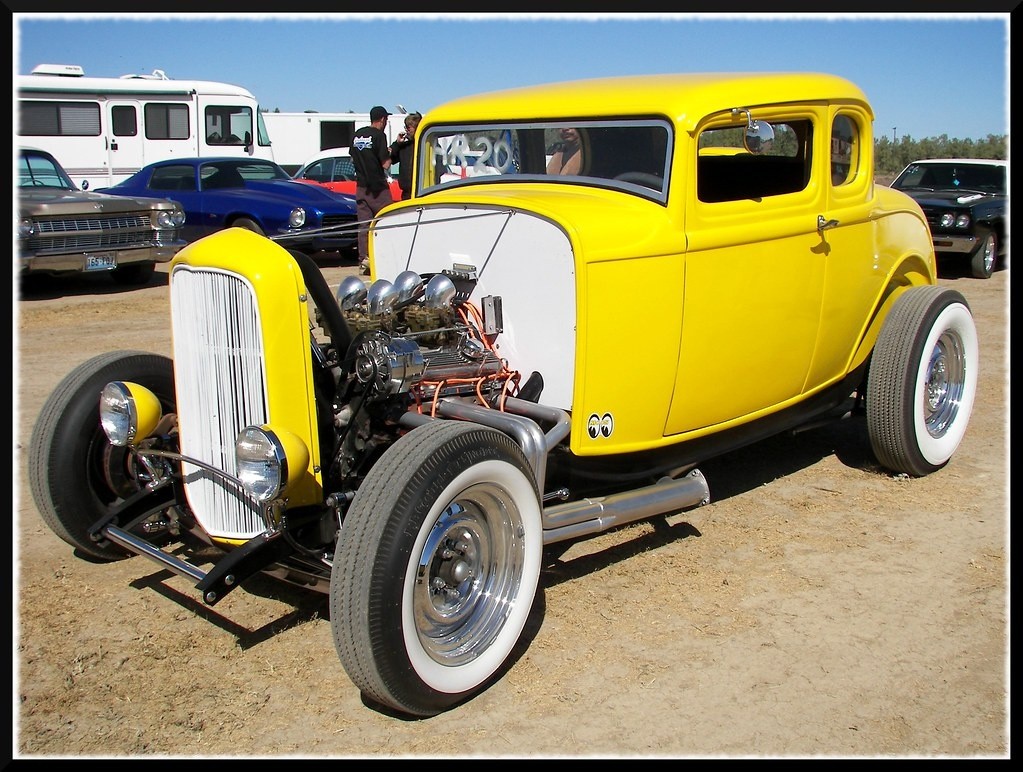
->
[305,174,355,182]
[698,154,805,202]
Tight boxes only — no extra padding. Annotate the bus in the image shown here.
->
[17,64,276,191]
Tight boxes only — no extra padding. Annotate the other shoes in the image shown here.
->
[359,257,370,275]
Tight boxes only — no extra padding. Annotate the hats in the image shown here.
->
[370,106,393,121]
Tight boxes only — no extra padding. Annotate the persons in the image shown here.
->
[546,128,583,177]
[348,106,394,275]
[388,115,446,201]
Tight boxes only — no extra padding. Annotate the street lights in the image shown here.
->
[892,127,896,176]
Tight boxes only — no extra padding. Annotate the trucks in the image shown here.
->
[167,108,470,179]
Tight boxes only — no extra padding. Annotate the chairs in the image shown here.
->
[977,166,1001,186]
[592,132,656,177]
[919,167,945,188]
[218,166,245,189]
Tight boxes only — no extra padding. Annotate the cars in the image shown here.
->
[889,158,1008,279]
[16,145,188,297]
[29,72,978,715]
[92,157,359,261]
[293,147,461,202]
[448,153,502,177]
